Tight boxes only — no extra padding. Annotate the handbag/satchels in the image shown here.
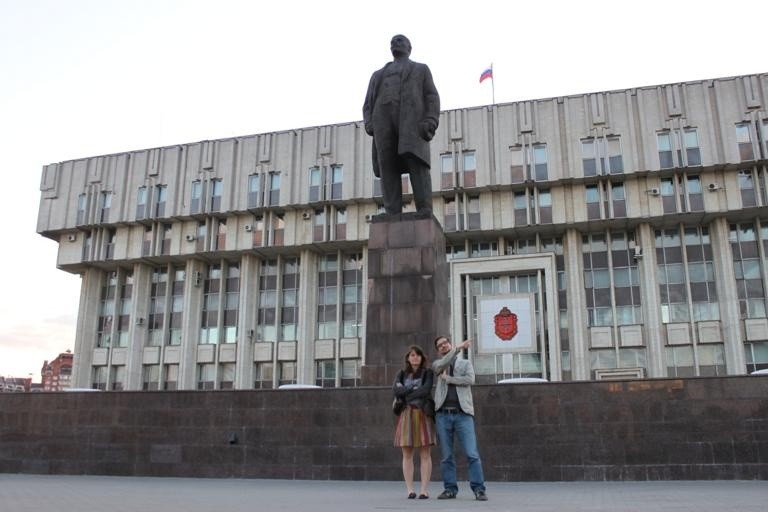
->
[392,396,404,416]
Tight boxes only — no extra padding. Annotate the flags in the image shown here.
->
[480,66,492,83]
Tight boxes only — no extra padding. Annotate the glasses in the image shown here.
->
[437,341,448,348]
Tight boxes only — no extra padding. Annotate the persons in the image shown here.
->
[363,35,440,215]
[393,345,440,498]
[432,336,488,500]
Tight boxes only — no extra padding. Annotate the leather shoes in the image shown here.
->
[438,490,456,498]
[439,409,463,414]
[475,491,487,500]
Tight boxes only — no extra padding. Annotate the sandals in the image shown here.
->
[408,492,429,499]
